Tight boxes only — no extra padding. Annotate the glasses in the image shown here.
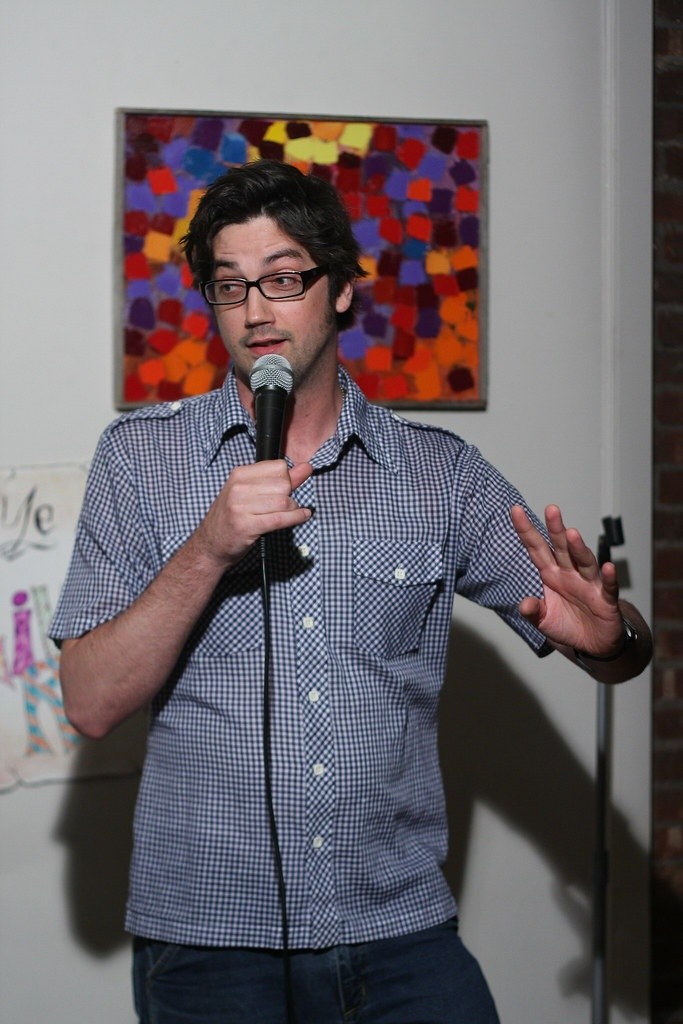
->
[199,265,329,305]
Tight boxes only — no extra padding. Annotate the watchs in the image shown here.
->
[578,617,637,661]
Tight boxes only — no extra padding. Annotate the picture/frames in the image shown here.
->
[112,106,492,413]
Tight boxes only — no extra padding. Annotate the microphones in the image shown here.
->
[249,353,296,463]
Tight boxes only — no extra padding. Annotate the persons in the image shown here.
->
[50,159,654,1024]
